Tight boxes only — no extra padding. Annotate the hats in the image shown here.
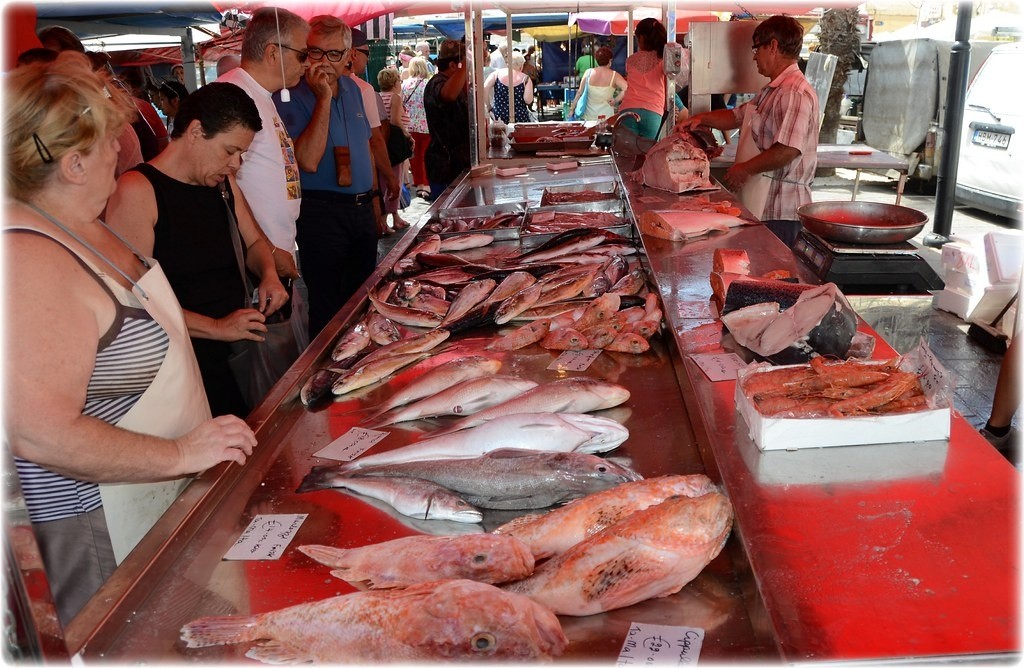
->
[351,28,368,47]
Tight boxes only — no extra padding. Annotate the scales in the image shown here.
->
[792,226,945,294]
[594,133,612,151]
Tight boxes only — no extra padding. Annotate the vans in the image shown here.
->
[954,37,1024,221]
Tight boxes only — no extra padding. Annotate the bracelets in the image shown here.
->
[272,246,276,253]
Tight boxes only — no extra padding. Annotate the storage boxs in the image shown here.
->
[734,360,951,451]
[933,231,1022,341]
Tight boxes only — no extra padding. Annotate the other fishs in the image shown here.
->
[178,204,754,663]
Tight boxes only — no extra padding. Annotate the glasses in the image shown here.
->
[751,41,770,53]
[357,49,370,58]
[275,44,309,63]
[398,79,403,84]
[306,46,346,62]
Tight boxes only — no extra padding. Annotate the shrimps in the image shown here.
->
[744,356,930,419]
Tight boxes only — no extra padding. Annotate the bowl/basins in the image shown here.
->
[797,201,929,245]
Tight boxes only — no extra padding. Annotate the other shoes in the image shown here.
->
[381,223,395,233]
[979,426,1019,452]
[394,221,410,230]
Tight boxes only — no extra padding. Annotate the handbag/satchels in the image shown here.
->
[227,317,298,407]
[574,68,592,117]
[386,124,412,167]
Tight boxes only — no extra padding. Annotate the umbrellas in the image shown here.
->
[568,9,729,35]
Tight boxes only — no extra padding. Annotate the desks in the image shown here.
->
[710,144,909,206]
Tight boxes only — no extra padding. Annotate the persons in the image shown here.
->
[394,33,542,205]
[3,62,257,631]
[617,19,735,144]
[977,330,1023,464]
[17,25,401,419]
[569,47,627,121]
[673,15,819,248]
